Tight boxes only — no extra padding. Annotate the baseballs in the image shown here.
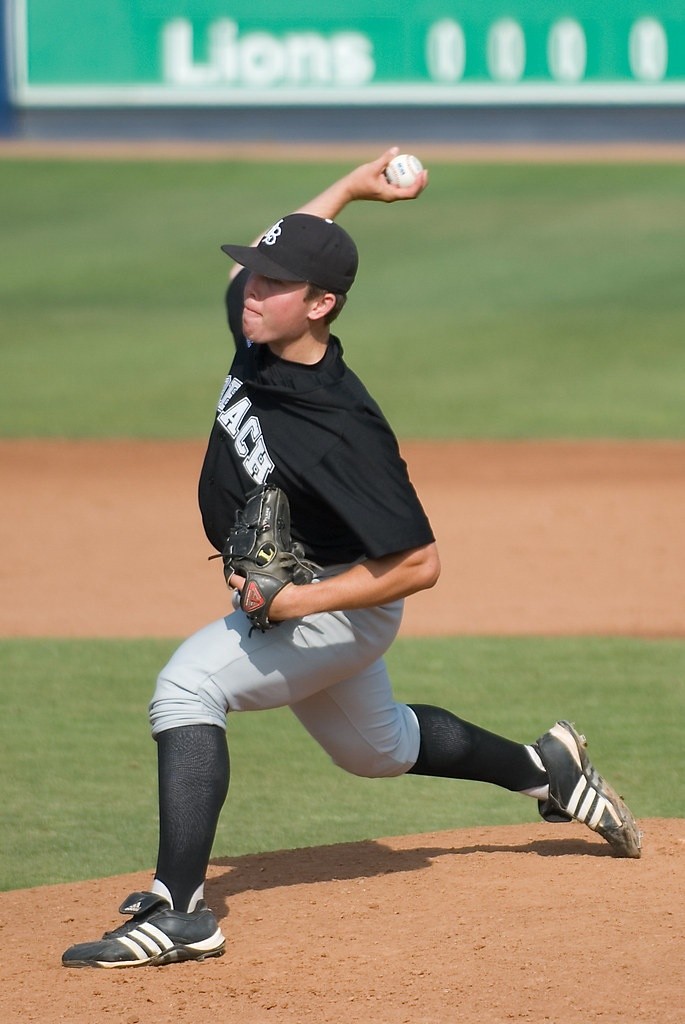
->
[385,154,424,189]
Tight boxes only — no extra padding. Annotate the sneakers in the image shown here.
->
[61,891,226,969]
[536,719,641,860]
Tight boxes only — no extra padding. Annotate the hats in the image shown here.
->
[220,213,358,295]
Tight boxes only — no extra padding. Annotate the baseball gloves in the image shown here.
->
[219,483,320,632]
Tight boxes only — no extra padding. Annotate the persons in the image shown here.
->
[62,147,641,968]
